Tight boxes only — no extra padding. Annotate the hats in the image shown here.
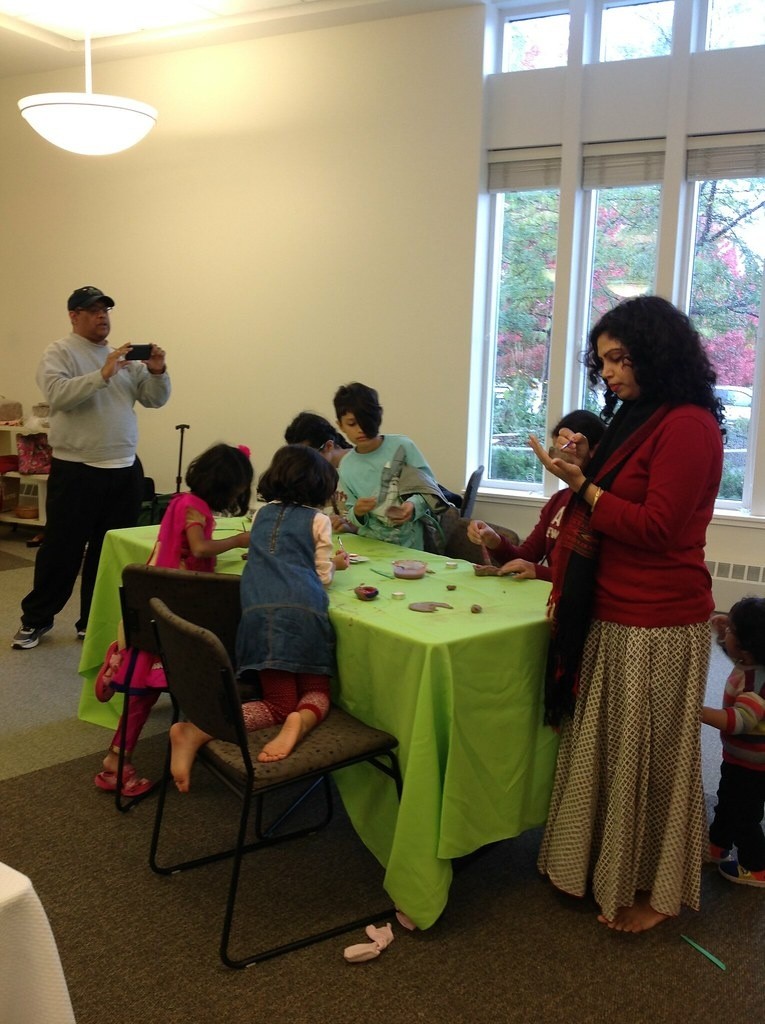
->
[67,286,115,311]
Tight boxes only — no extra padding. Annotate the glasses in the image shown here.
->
[73,303,114,314]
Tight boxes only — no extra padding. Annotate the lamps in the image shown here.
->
[18,8,156,157]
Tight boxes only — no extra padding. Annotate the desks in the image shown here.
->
[77,511,559,930]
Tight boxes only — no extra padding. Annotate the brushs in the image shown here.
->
[475,522,492,566]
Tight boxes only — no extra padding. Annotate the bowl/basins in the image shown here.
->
[392,560,427,579]
[13,506,38,519]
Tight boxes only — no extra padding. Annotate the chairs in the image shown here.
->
[149,597,402,967]
[115,561,325,837]
[457,467,487,520]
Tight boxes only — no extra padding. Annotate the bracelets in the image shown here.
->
[577,476,592,497]
[592,487,602,511]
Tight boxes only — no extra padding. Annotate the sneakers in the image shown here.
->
[77,626,86,640]
[10,617,55,650]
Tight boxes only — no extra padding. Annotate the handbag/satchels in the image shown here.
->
[15,433,53,474]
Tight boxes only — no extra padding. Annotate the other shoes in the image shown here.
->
[706,844,731,862]
[719,860,765,888]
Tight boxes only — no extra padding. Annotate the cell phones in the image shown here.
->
[125,345,151,361]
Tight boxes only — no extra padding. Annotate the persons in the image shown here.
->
[284,383,439,555]
[93,444,253,797]
[170,442,350,793]
[11,285,171,651]
[529,296,727,932]
[699,597,765,888]
[467,409,607,585]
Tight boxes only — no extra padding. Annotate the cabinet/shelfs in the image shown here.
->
[0,426,49,526]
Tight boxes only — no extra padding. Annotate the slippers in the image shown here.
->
[95,641,116,704]
[95,766,152,796]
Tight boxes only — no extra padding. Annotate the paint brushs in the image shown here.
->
[338,536,344,552]
[560,441,572,450]
[242,522,246,533]
